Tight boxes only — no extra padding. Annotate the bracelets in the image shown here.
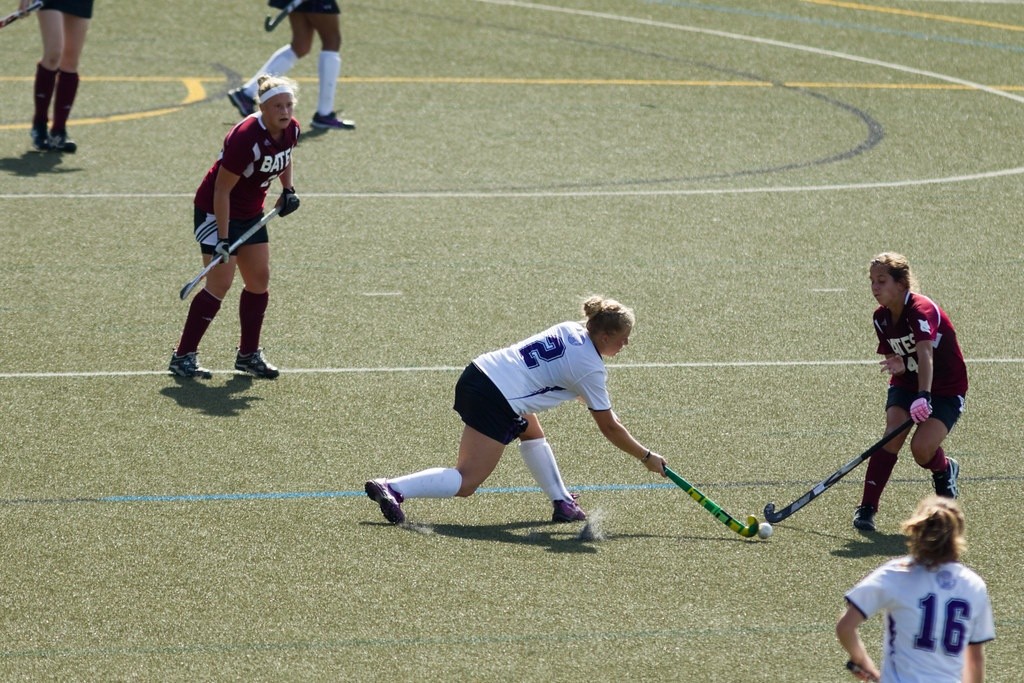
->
[641,451,650,463]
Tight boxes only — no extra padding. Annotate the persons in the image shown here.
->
[15,0,94,154]
[852,250,969,532]
[169,76,300,381]
[228,0,356,131]
[836,494,996,683]
[364,295,668,526]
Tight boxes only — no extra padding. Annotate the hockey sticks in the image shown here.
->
[764,404,934,523]
[847,661,878,682]
[0,1,43,29]
[178,206,281,301]
[663,465,759,537]
[264,0,304,32]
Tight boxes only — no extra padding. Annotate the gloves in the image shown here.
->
[910,391,933,424]
[274,186,299,217]
[212,238,230,264]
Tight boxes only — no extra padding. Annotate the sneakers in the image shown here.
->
[853,506,876,533]
[550,496,586,521]
[29,126,53,150]
[168,352,214,381]
[364,478,404,524]
[226,87,257,118]
[931,457,959,498]
[235,349,280,379]
[48,129,77,153]
[309,108,355,131]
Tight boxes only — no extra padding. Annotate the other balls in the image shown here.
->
[758,522,772,539]
